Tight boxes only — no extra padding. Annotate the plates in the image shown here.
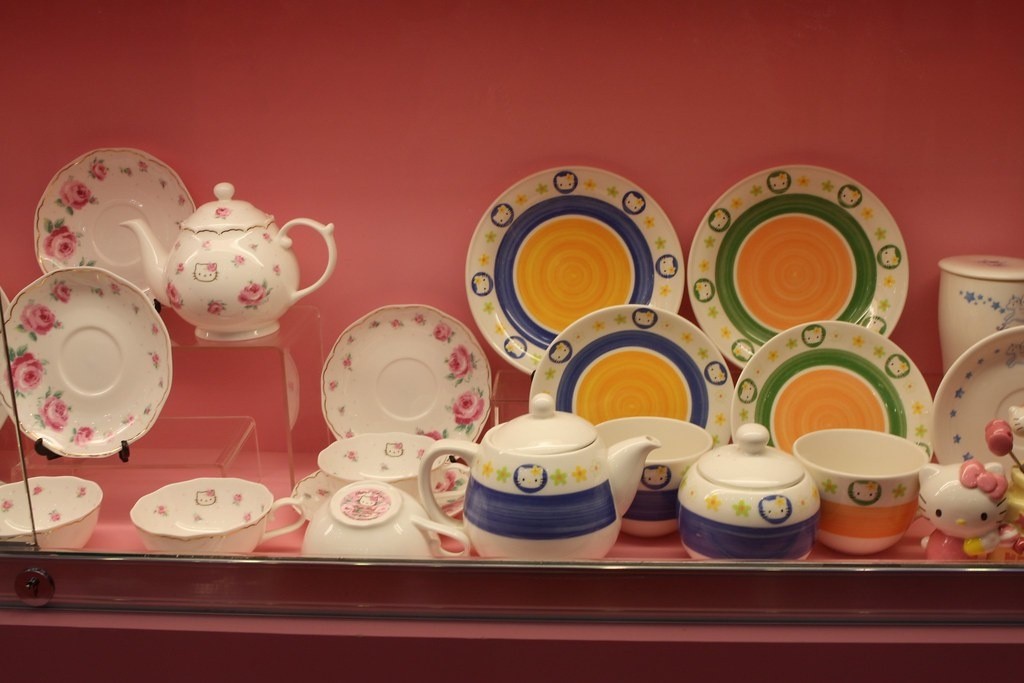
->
[0,286,10,430]
[933,326,1024,464]
[529,304,731,444]
[320,304,493,460]
[466,165,684,374]
[291,462,470,522]
[34,147,196,300]
[686,164,910,370]
[0,266,173,458]
[732,321,932,464]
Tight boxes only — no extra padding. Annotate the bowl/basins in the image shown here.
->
[0,476,103,549]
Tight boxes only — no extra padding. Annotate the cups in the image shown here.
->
[131,477,308,555]
[937,255,1024,376]
[594,417,712,537]
[679,424,819,562]
[793,429,929,554]
[317,433,470,504]
[303,479,470,560]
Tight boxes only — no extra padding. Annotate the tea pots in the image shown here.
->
[119,183,337,342]
[417,391,660,561]
